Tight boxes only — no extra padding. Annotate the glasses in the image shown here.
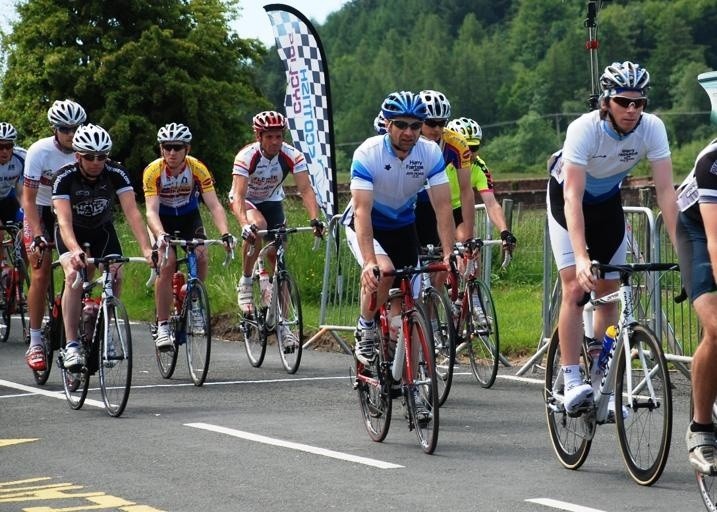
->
[162,143,185,152]
[58,128,76,134]
[79,153,108,161]
[0,143,15,150]
[608,94,647,108]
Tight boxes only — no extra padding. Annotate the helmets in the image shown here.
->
[157,122,193,143]
[0,122,18,140]
[47,99,87,128]
[252,111,288,142]
[71,123,112,155]
[373,89,482,141]
[599,60,650,90]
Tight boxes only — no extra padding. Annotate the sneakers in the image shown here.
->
[595,393,629,423]
[564,383,593,418]
[433,329,448,357]
[61,346,85,373]
[470,306,493,336]
[189,309,205,338]
[154,329,173,352]
[281,328,300,353]
[401,397,432,427]
[354,321,378,367]
[102,337,119,368]
[236,278,255,314]
[685,419,717,477]
[25,345,48,371]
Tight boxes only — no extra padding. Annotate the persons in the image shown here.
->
[676,71,717,473]
[227,110,326,350]
[1,98,162,368]
[342,90,518,426]
[143,123,237,353]
[546,65,680,419]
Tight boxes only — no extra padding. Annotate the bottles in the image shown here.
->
[83,297,95,335]
[596,325,618,371]
[453,290,464,316]
[259,269,269,300]
[386,315,401,362]
[172,270,185,312]
[52,293,61,317]
